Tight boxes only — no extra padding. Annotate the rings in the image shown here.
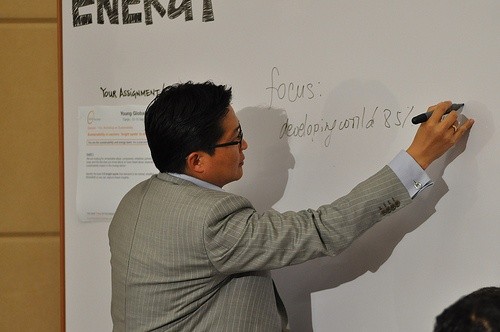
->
[451,124,457,132]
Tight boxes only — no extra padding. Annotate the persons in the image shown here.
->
[107,81,474,332]
[433,286,500,332]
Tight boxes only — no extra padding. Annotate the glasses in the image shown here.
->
[182,123,244,159]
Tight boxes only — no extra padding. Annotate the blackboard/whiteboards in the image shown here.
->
[57,0,499,331]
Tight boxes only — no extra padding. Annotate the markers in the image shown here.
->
[411,103,465,124]
